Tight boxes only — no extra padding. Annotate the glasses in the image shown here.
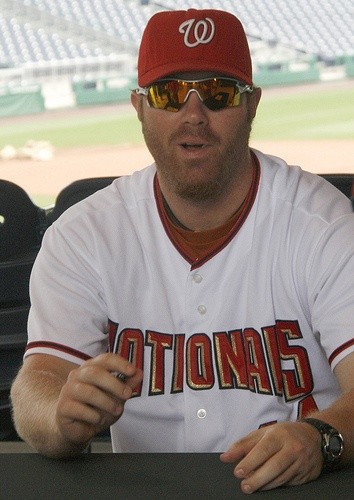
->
[134,78,252,112]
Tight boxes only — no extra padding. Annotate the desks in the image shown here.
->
[0,452,353,500]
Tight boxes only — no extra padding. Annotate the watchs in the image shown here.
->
[304,418,344,468]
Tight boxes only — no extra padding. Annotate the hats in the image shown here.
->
[139,7,254,88]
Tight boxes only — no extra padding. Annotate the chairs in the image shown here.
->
[0,176,119,429]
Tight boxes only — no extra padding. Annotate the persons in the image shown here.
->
[10,8,354,493]
